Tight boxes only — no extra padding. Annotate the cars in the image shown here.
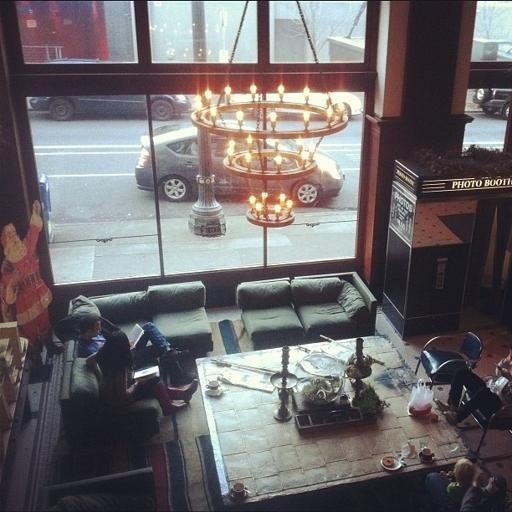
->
[135,120,344,208]
[473,88,510,119]
[29,57,190,122]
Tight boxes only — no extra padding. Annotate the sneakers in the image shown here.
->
[434,398,458,426]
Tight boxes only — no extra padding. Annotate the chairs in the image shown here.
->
[458,374,512,453]
[415,331,483,390]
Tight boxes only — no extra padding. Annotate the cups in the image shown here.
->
[206,381,219,391]
[421,447,432,457]
[233,483,244,497]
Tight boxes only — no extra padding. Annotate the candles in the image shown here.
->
[282,345,289,365]
[355,337,364,356]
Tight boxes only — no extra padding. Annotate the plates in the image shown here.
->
[380,456,402,471]
[205,390,223,397]
[227,490,249,503]
[419,452,435,463]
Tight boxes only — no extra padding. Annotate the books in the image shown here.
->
[126,322,145,350]
[133,364,160,383]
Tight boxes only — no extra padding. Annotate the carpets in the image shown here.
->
[22,320,474,512]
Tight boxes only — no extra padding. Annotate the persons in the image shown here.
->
[443,457,484,505]
[83,331,199,418]
[433,347,512,427]
[75,311,197,386]
[423,470,507,512]
[0,199,65,369]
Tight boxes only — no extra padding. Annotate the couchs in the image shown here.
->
[236,272,378,350]
[59,280,214,436]
[37,466,158,512]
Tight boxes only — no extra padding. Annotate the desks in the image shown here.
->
[0,336,32,512]
[195,334,468,506]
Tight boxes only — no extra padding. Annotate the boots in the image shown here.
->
[153,379,197,416]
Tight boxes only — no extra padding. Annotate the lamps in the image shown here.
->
[191,0,349,227]
